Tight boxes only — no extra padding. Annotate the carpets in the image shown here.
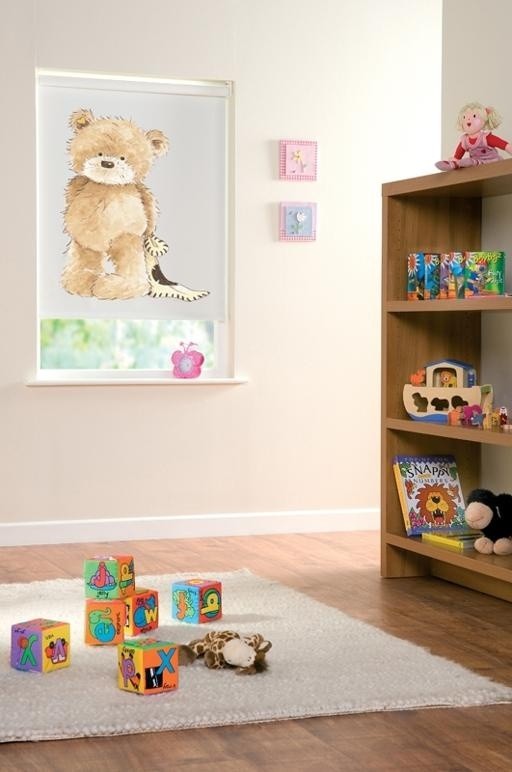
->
[0,569,512,743]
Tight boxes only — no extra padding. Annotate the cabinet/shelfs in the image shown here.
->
[382,158,512,604]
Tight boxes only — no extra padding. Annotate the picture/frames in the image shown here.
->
[276,200,318,243]
[280,138,318,179]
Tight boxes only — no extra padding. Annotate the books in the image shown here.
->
[392,454,470,537]
[421,533,485,549]
[407,250,506,300]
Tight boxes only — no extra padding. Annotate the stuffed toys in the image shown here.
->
[63,108,211,300]
[184,631,273,676]
[464,487,512,556]
[435,102,512,172]
